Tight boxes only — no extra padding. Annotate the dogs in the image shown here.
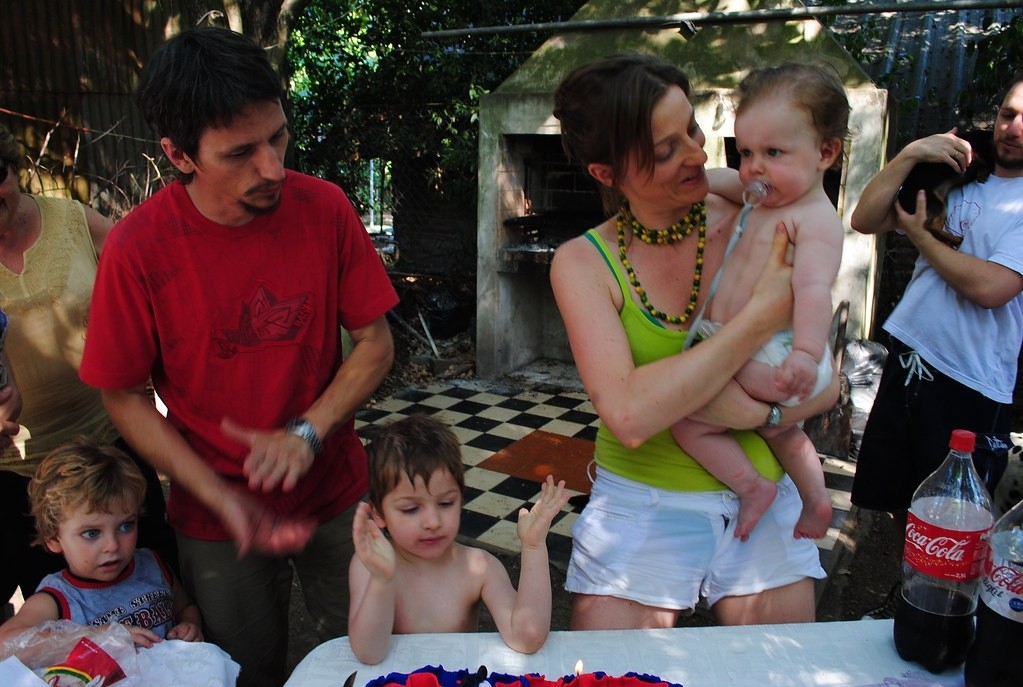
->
[899,129,996,249]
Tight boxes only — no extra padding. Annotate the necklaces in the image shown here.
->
[616,198,707,323]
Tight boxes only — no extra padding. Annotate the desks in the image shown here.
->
[284,617,977,687]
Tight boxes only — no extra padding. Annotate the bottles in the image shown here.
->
[893,429,996,676]
[964,500,1023,687]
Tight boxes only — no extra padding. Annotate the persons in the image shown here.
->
[0,126,166,629]
[551,53,843,631]
[672,62,854,542]
[852,77,1022,621]
[0,432,204,671]
[80,27,400,687]
[347,413,571,664]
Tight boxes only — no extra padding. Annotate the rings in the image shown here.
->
[953,151,958,156]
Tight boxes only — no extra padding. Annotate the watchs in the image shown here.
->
[765,404,782,428]
[287,419,321,454]
